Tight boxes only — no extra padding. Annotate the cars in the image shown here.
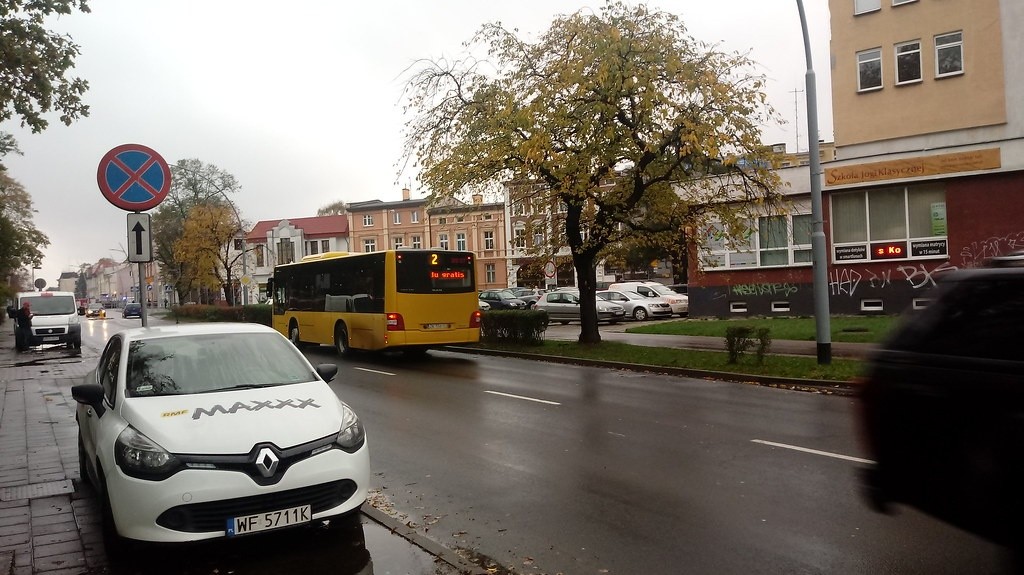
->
[476,297,490,310]
[85,303,106,317]
[123,303,142,319]
[511,289,547,311]
[71,320,372,547]
[849,265,1024,554]
[532,289,626,325]
[478,289,527,310]
[595,289,673,320]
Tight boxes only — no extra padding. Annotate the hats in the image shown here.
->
[23,301,32,306]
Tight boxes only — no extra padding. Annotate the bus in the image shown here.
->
[265,246,482,360]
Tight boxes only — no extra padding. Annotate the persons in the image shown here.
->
[17,301,34,354]
[164,298,167,308]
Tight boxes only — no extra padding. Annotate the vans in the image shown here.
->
[7,291,82,349]
[608,281,689,317]
